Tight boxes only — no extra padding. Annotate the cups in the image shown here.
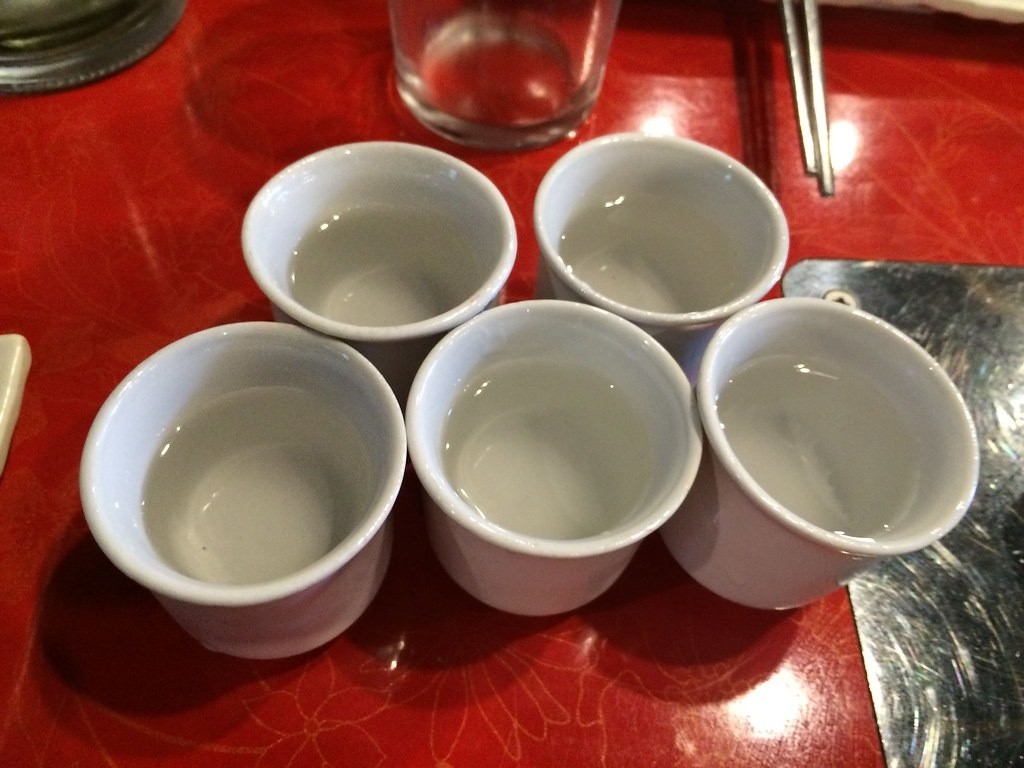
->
[0,0,186,94]
[240,139,517,408]
[386,0,621,150]
[79,319,407,662]
[658,296,980,609]
[405,298,704,616]
[532,133,789,393]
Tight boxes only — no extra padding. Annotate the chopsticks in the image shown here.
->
[776,0,835,199]
